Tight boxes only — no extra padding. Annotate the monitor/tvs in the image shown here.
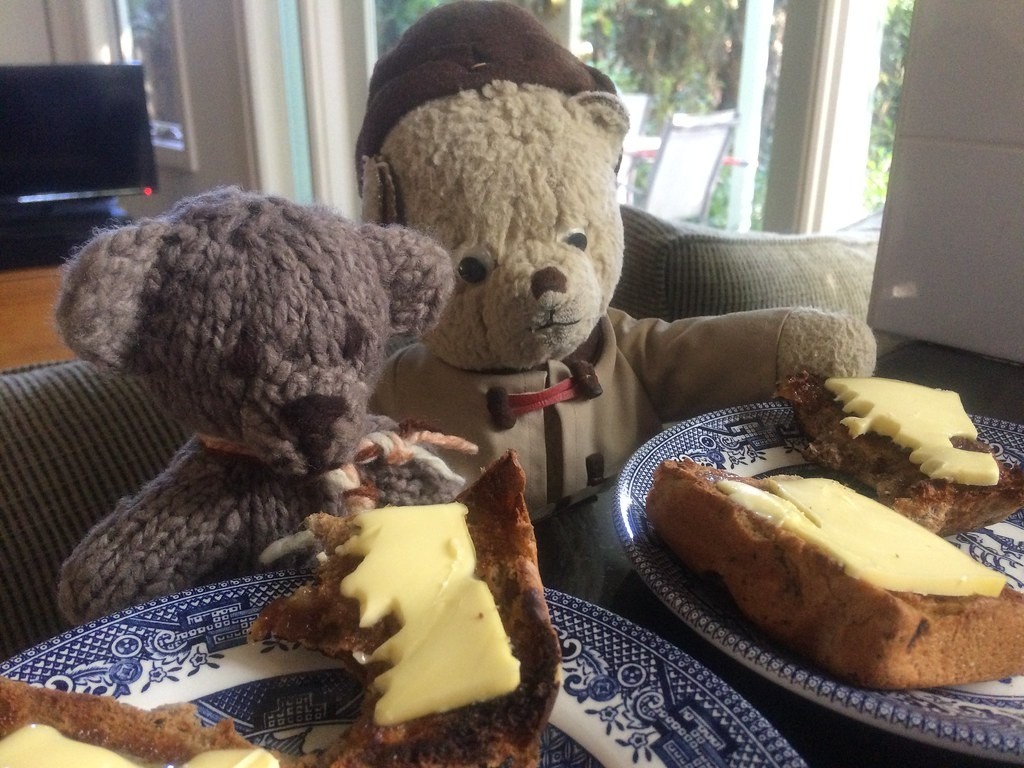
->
[0,64,160,239]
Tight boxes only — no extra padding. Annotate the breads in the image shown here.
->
[772,369,1024,537]
[245,449,563,768]
[648,458,1024,689]
[0,677,308,768]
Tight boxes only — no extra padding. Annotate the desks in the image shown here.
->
[616,135,748,203]
[532,338,1024,768]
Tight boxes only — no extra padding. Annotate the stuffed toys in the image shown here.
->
[357,1,876,512]
[58,187,479,625]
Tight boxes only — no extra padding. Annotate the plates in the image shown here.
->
[612,397,1024,762]
[0,567,809,768]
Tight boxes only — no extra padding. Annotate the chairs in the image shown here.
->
[617,93,648,204]
[637,110,736,226]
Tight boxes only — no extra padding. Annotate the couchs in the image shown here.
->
[0,203,882,665]
